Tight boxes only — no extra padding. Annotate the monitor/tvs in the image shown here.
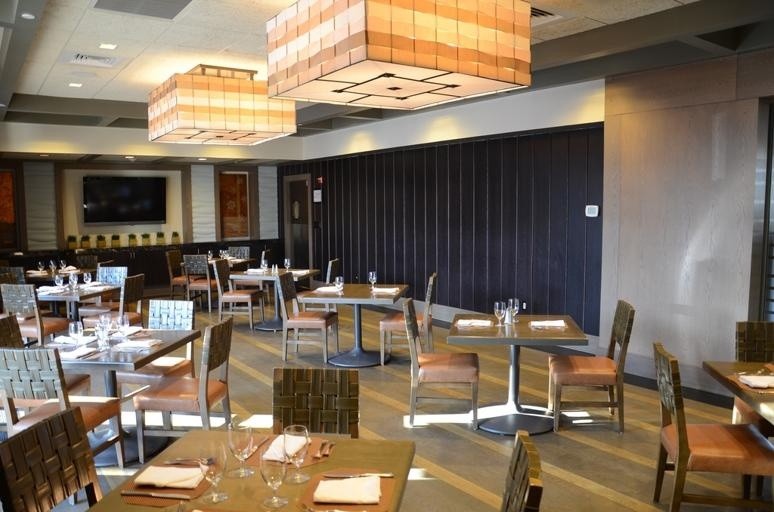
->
[82,176,166,227]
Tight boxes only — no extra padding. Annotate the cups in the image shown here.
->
[271,263,278,275]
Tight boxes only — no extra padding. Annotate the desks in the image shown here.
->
[703,362,773,427]
[87,430,418,512]
[303,283,410,370]
[446,313,588,437]
[229,268,319,331]
[43,325,201,476]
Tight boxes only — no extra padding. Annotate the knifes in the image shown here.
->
[245,437,267,458]
[121,487,191,503]
[321,471,394,480]
[75,348,98,359]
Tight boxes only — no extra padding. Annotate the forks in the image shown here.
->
[293,502,339,511]
[312,440,335,460]
[164,456,214,467]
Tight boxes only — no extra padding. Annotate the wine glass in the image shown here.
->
[222,418,259,479]
[33,259,69,274]
[70,312,132,351]
[283,258,290,273]
[198,442,228,505]
[492,300,507,328]
[282,423,310,484]
[206,249,228,261]
[260,260,268,275]
[259,446,290,510]
[335,276,344,296]
[368,270,377,292]
[54,272,90,292]
[508,297,520,324]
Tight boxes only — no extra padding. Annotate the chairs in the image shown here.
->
[548,300,637,435]
[2,247,266,347]
[300,258,340,312]
[1,406,104,511]
[133,315,232,464]
[402,297,480,431]
[275,272,340,364]
[2,346,126,506]
[116,298,195,430]
[652,342,773,509]
[731,320,774,442]
[499,429,544,510]
[379,272,438,366]
[272,369,358,439]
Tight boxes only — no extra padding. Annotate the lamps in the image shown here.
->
[262,0,532,112]
[146,62,297,146]
